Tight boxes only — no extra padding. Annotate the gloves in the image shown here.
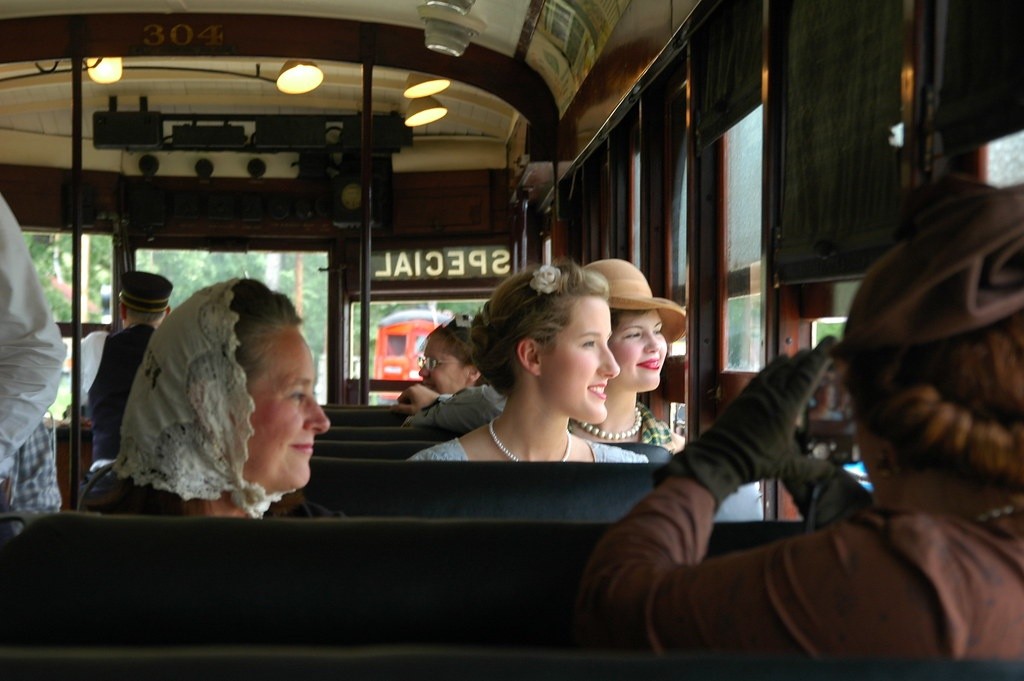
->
[779,428,872,532]
[651,335,837,517]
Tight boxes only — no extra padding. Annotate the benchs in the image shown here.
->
[0,512,808,651]
[314,427,472,442]
[310,439,445,459]
[324,408,411,427]
[74,460,767,520]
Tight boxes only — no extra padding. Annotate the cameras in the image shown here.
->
[802,364,858,465]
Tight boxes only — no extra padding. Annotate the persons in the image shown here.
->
[418,315,489,394]
[575,174,1024,659]
[809,382,852,420]
[393,383,507,433]
[0,195,65,542]
[408,263,650,462]
[567,259,685,455]
[82,271,174,478]
[82,280,343,519]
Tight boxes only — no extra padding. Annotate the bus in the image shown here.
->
[372,309,456,405]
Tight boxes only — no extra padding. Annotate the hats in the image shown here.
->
[582,258,687,344]
[828,170,1023,361]
[118,272,174,313]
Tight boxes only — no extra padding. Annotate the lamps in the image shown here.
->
[405,96,448,126]
[403,72,451,99]
[417,5,487,57]
[276,59,324,94]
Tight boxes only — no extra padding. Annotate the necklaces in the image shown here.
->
[489,416,572,462]
[576,408,642,439]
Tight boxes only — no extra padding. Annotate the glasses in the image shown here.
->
[419,357,466,371]
